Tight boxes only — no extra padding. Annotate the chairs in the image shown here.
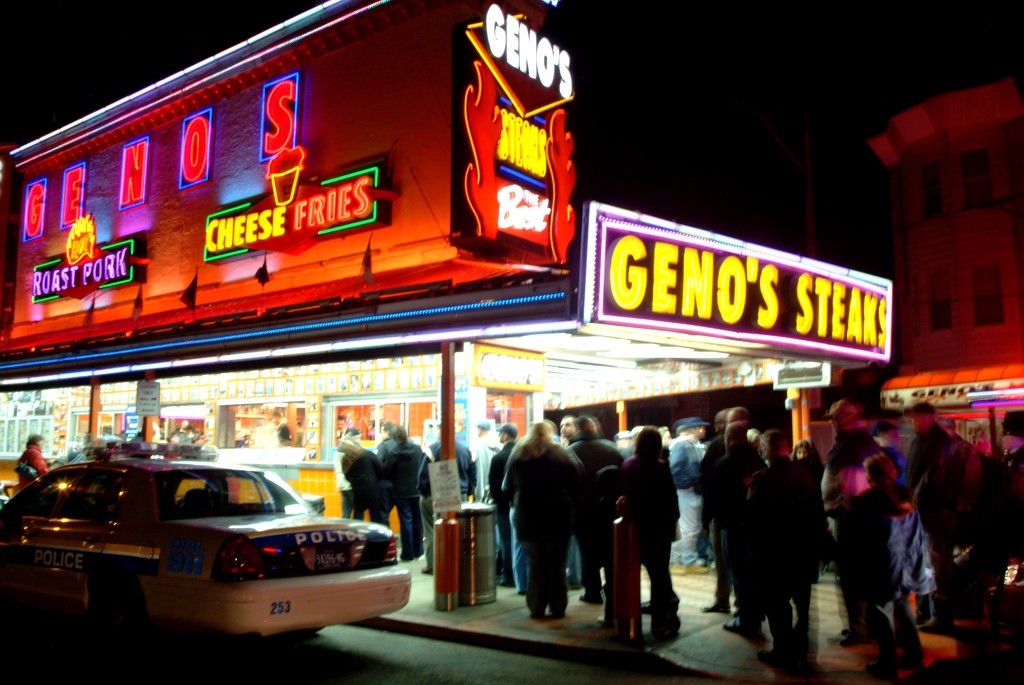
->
[183,488,219,509]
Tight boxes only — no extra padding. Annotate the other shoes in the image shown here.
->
[421,567,433,575]
[580,594,594,603]
[641,600,651,613]
[895,658,924,670]
[703,604,730,614]
[674,564,709,575]
[723,619,744,634]
[840,631,858,646]
[758,648,791,666]
[916,621,950,635]
[865,662,896,679]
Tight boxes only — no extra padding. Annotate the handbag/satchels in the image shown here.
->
[14,461,39,481]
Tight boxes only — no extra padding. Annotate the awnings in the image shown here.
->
[159,406,211,420]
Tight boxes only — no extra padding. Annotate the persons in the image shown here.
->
[333,415,504,575]
[167,418,205,447]
[695,406,837,669]
[268,409,305,448]
[66,440,83,462]
[100,424,123,441]
[237,434,250,449]
[619,429,682,643]
[869,402,1024,638]
[614,415,716,574]
[16,433,49,489]
[487,412,625,625]
[818,398,939,681]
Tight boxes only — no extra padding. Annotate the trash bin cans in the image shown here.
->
[433,502,496,606]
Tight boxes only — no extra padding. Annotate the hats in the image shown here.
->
[824,399,858,418]
[685,416,709,426]
[496,423,518,438]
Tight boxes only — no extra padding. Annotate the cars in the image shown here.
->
[0,460,410,649]
